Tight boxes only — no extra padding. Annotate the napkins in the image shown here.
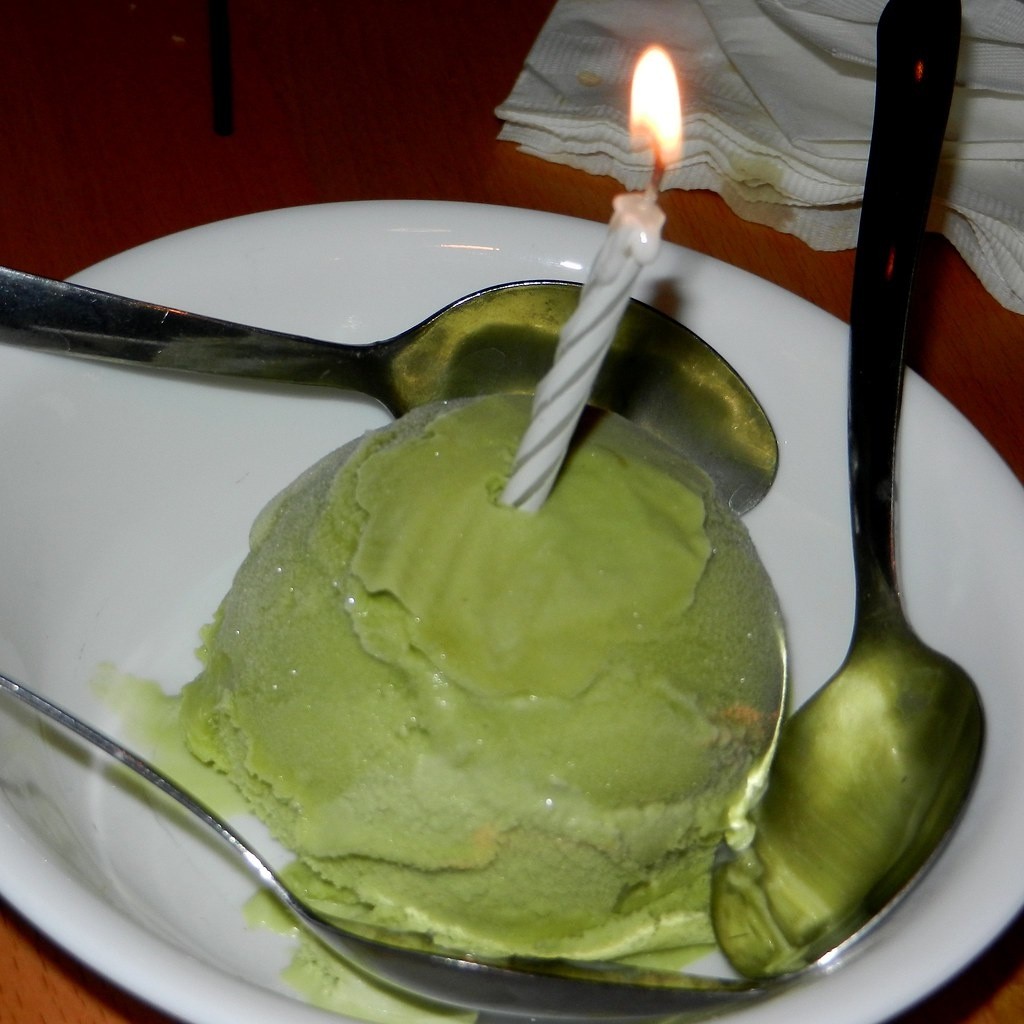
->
[493,0,1024,315]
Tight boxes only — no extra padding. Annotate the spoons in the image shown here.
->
[708,0,987,985]
[0,257,779,520]
[0,672,785,1024]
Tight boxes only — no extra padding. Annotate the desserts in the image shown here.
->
[185,394,787,964]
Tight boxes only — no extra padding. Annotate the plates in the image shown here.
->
[0,199,1024,1024]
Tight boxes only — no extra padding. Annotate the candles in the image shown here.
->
[500,31,683,510]
